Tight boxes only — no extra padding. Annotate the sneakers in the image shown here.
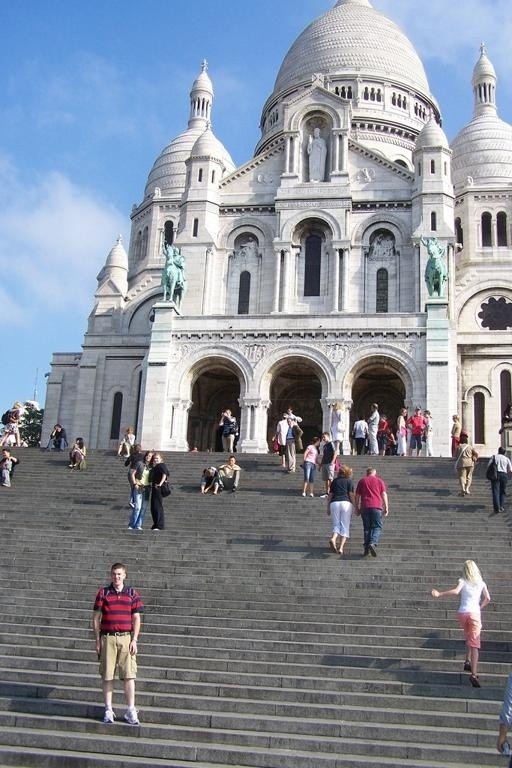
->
[123,709,140,725]
[368,543,377,558]
[319,493,330,498]
[329,539,337,553]
[364,548,369,556]
[468,673,481,689]
[463,661,471,672]
[103,709,115,723]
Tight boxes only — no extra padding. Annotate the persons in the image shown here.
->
[306,126,328,181]
[450,414,478,497]
[420,233,447,281]
[45,424,69,452]
[370,233,383,255]
[496,673,512,768]
[0,396,28,489]
[91,562,145,726]
[189,408,243,495]
[161,242,185,290]
[272,402,435,557]
[246,237,259,258]
[117,427,172,532]
[431,559,491,687]
[485,446,512,514]
[69,437,87,471]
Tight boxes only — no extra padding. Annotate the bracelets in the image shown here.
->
[132,638,138,642]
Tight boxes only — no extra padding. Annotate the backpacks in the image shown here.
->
[486,460,498,481]
[335,458,340,473]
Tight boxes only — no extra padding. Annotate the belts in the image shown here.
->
[102,632,131,636]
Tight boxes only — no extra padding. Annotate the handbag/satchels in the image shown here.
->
[230,423,239,434]
[1,412,11,425]
[159,480,171,498]
[454,462,459,474]
